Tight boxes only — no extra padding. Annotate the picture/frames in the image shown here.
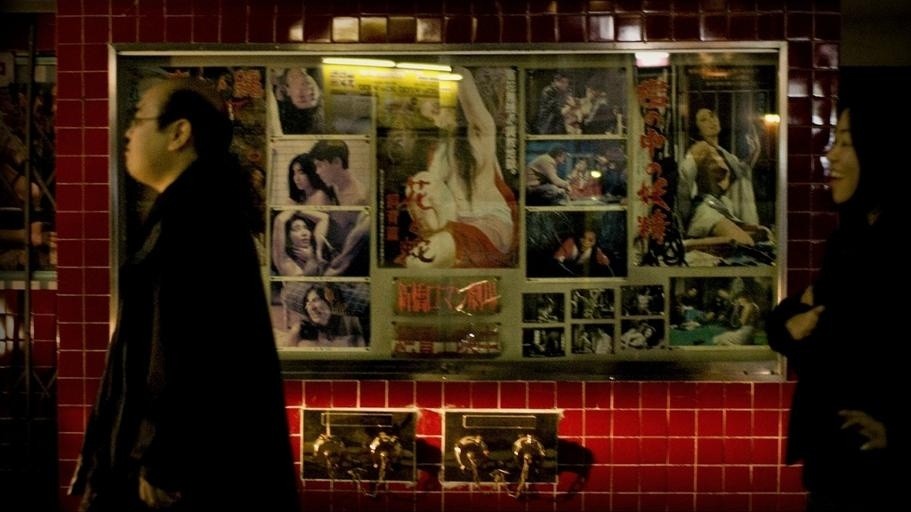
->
[108,39,789,384]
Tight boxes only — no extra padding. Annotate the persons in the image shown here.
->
[762,89,908,512]
[66,79,302,512]
